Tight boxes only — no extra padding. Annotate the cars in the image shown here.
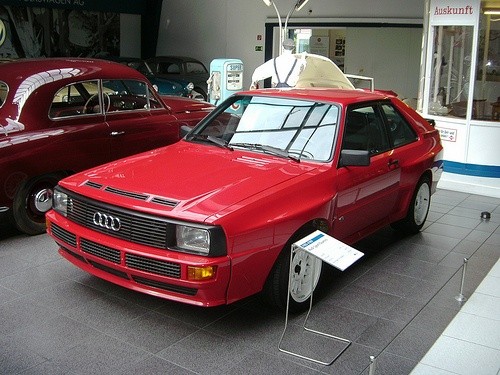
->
[119,55,210,102]
[43,87,446,314]
[0,57,242,236]
